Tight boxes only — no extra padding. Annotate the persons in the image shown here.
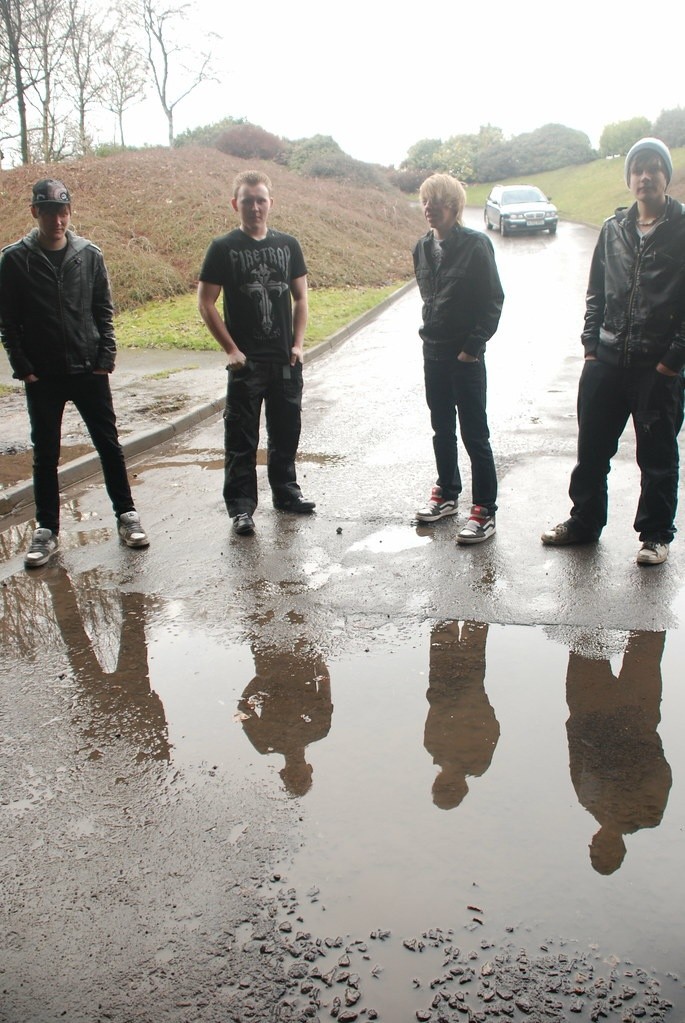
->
[34,550,174,797]
[563,565,668,876]
[410,172,506,544]
[419,548,505,814]
[195,169,317,533]
[222,539,336,800]
[1,175,150,569]
[540,136,685,564]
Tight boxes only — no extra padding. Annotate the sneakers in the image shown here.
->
[541,517,600,544]
[23,528,60,567]
[416,487,459,521]
[117,511,150,547]
[455,504,496,543]
[637,535,670,563]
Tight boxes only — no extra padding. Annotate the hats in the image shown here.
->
[28,178,71,205]
[624,138,673,190]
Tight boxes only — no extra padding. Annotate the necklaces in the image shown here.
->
[633,199,669,226]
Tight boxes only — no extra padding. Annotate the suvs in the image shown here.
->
[483,183,559,235]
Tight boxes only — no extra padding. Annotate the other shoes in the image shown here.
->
[274,493,315,511]
[232,513,255,533]
